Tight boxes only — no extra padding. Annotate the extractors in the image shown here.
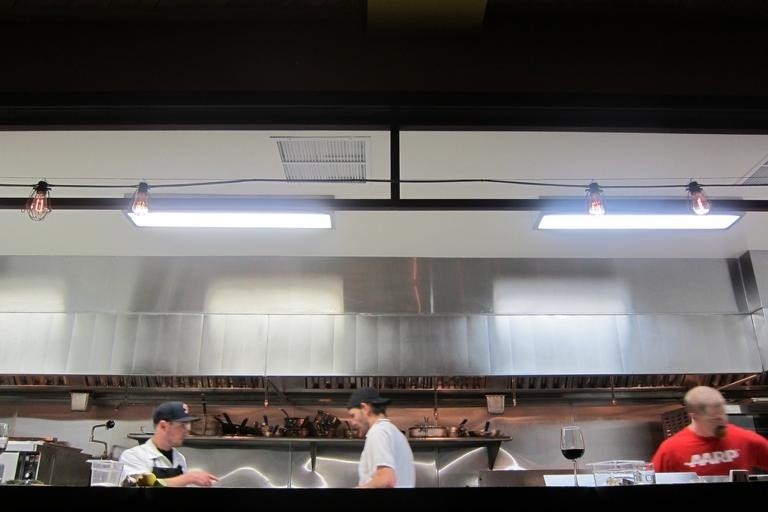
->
[0,248,767,396]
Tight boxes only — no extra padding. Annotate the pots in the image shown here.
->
[183,397,502,440]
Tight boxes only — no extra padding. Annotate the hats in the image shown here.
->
[347,388,391,408]
[154,401,201,424]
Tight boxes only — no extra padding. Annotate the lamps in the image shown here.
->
[119,193,334,233]
[1,179,767,219]
[533,196,743,232]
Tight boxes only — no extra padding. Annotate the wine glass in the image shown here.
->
[561,424,586,486]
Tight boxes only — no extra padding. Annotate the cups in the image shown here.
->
[0,421,11,456]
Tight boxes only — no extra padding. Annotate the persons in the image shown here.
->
[346,386,417,489]
[642,385,767,476]
[116,400,219,490]
